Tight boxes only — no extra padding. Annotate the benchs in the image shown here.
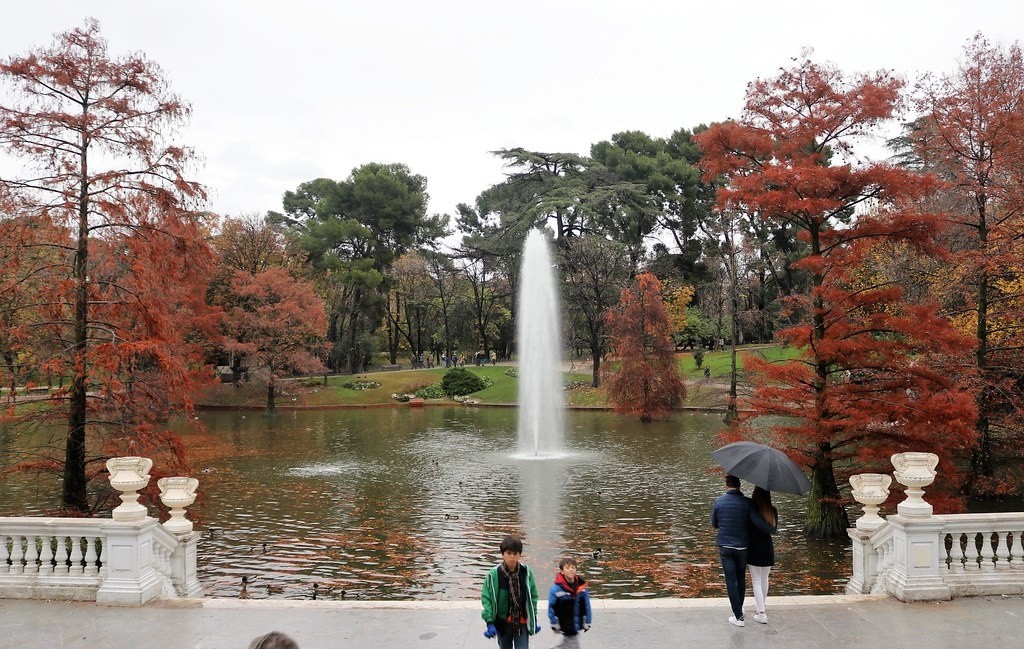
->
[317,370,334,376]
[381,364,400,371]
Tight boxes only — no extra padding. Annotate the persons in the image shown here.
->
[481,537,541,649]
[711,476,773,627]
[704,366,710,378]
[452,354,459,367]
[459,354,465,367]
[248,631,298,649]
[491,350,496,365]
[411,352,434,370]
[548,558,591,649]
[442,354,446,368]
[475,352,484,366]
[746,485,778,624]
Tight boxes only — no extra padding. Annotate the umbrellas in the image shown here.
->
[710,441,812,495]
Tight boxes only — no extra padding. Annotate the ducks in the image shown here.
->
[593,548,602,557]
[241,543,360,598]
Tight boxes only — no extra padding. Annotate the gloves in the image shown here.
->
[552,626,564,634]
[535,625,541,633]
[484,624,497,640]
[582,624,590,632]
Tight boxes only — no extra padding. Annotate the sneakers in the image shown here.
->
[754,614,767,624]
[728,616,745,627]
